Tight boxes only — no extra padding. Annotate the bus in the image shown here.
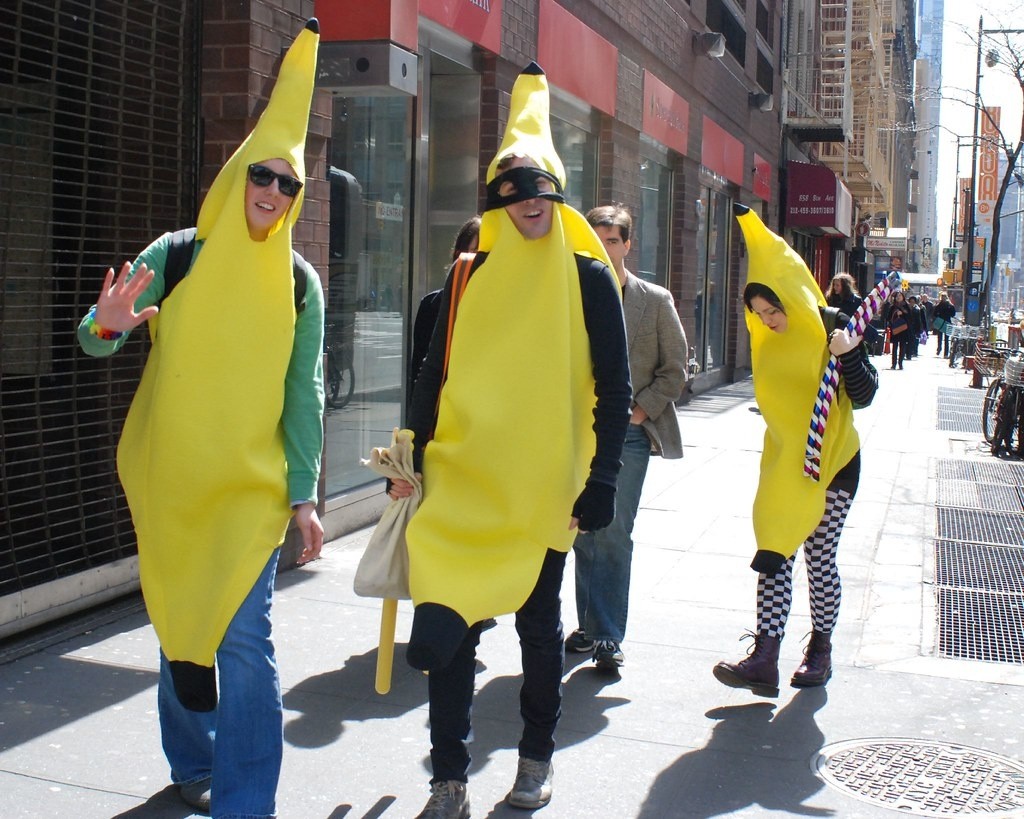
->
[901,272,944,302]
[326,166,363,377]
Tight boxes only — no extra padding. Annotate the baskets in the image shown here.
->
[974,342,1013,376]
[1003,355,1024,388]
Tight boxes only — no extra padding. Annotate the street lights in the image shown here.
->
[990,286,1024,308]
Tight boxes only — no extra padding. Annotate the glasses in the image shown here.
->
[249,163,305,197]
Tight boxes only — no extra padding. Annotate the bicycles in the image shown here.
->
[946,316,986,367]
[973,342,1024,459]
[323,330,355,409]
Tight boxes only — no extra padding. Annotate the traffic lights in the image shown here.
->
[1006,268,1011,276]
[937,278,944,286]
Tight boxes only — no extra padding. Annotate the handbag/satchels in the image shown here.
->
[892,315,907,334]
[920,331,927,344]
[933,318,950,334]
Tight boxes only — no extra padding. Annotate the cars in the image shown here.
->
[991,309,1024,324]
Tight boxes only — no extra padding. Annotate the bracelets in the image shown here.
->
[89,319,123,340]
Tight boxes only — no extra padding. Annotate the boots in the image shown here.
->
[790,631,833,687]
[713,631,782,698]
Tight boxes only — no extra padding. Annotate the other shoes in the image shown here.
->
[899,362,903,370]
[936,347,942,355]
[891,363,896,369]
[179,777,211,811]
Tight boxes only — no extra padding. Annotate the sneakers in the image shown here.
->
[509,754,553,809]
[564,626,594,651]
[417,782,471,819]
[591,637,623,669]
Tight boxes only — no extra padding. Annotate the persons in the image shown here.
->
[559,206,690,668]
[385,133,632,819]
[76,132,326,819]
[714,266,879,697]
[827,273,957,370]
[407,216,498,635]
